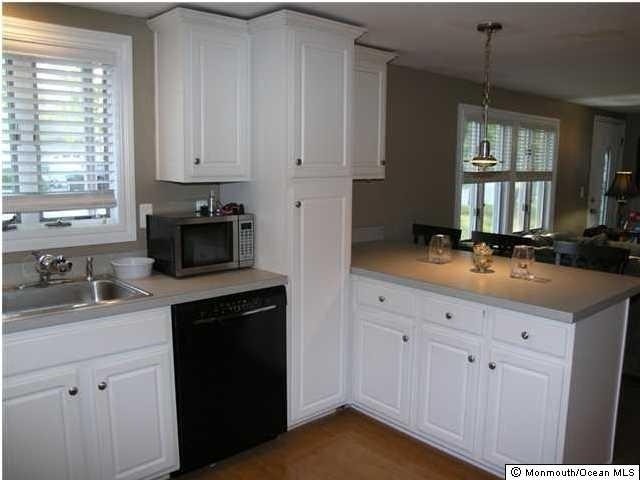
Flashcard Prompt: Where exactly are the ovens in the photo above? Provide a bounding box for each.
[171,286,287,473]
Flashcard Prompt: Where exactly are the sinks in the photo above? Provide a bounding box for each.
[3,276,152,319]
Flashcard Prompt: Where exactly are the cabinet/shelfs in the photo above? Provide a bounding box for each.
[413,275,640,480]
[216,181,354,431]
[350,266,420,438]
[2,297,180,480]
[246,7,370,181]
[350,43,400,180]
[145,6,251,185]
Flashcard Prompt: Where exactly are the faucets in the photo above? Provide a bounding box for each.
[37,252,74,287]
[84,256,95,282]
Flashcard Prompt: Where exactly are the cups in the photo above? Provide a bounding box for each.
[427,234,452,263]
[510,245,535,281]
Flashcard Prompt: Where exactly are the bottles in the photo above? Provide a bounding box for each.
[208,189,216,216]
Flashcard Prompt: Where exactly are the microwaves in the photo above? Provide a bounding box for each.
[146,214,256,277]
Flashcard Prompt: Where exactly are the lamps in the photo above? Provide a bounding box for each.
[462,21,504,168]
[604,170,640,230]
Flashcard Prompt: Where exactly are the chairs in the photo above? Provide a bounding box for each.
[412,223,463,250]
[551,239,631,275]
[470,229,536,260]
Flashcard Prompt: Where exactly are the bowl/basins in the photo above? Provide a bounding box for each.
[110,257,155,279]
